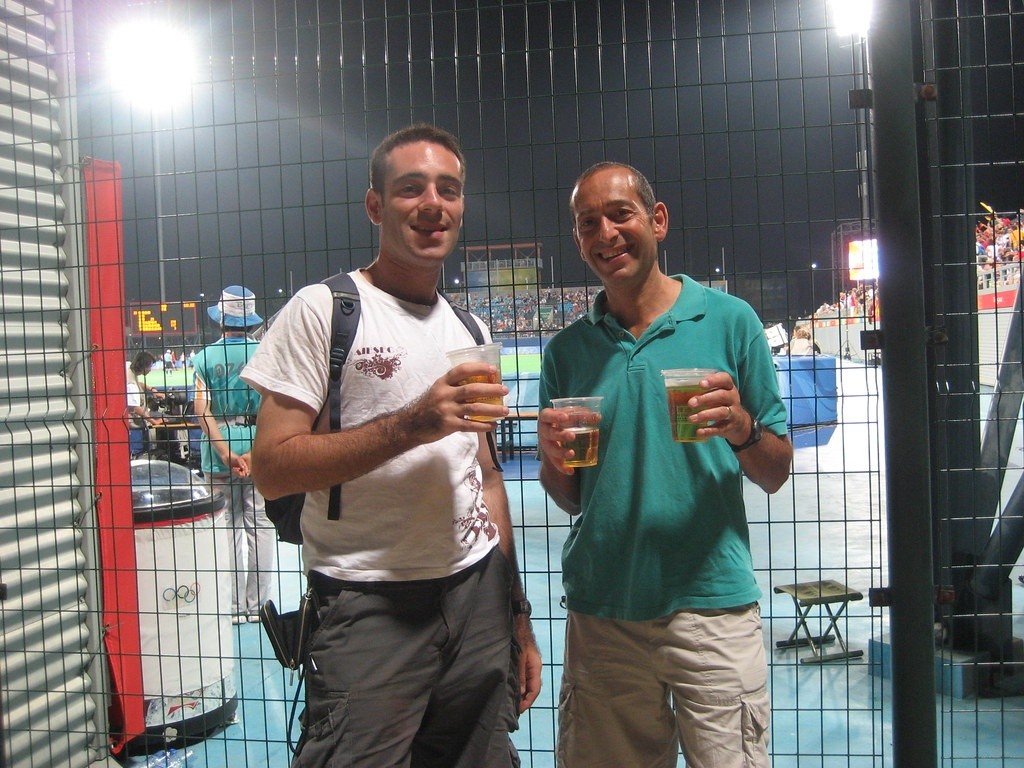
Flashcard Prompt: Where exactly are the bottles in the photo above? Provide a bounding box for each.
[131,748,178,768]
[153,749,194,768]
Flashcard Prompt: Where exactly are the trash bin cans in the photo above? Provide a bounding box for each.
[127,458,241,757]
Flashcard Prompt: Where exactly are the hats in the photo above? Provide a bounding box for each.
[207,286,263,327]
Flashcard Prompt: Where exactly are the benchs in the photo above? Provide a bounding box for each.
[498,411,540,460]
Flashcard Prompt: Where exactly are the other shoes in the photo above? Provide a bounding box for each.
[232,613,261,624]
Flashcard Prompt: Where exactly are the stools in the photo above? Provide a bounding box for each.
[773,579,863,663]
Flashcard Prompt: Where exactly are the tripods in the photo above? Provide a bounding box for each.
[833,289,864,362]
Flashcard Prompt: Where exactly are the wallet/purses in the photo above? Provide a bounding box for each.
[261,592,312,687]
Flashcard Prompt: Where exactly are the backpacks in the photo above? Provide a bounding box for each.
[263,269,504,544]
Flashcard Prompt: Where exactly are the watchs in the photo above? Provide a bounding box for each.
[514,598,533,617]
[725,415,762,452]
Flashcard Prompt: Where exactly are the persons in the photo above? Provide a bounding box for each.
[123,213,1024,623]
[238,123,544,768]
[538,163,794,768]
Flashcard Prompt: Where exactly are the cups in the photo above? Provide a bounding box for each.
[661,368,719,443]
[550,396,604,468]
[447,342,506,422]
